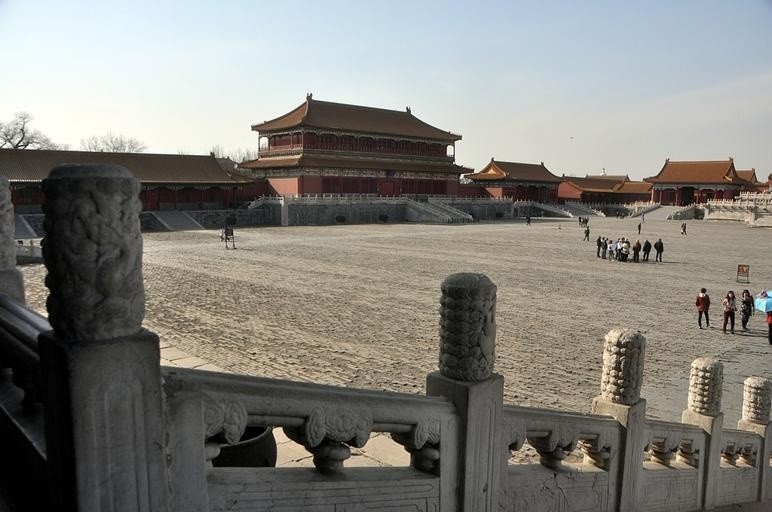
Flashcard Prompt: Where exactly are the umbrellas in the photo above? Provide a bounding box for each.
[755,290,772,314]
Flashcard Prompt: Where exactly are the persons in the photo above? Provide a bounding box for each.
[766,311,772,346]
[619,212,622,218]
[637,222,642,235]
[740,289,754,332]
[524,213,533,226]
[578,215,590,242]
[695,287,711,329]
[680,223,687,235]
[616,211,620,219]
[680,223,684,233]
[595,236,665,264]
[642,213,645,222]
[721,290,738,334]
[620,212,625,219]
